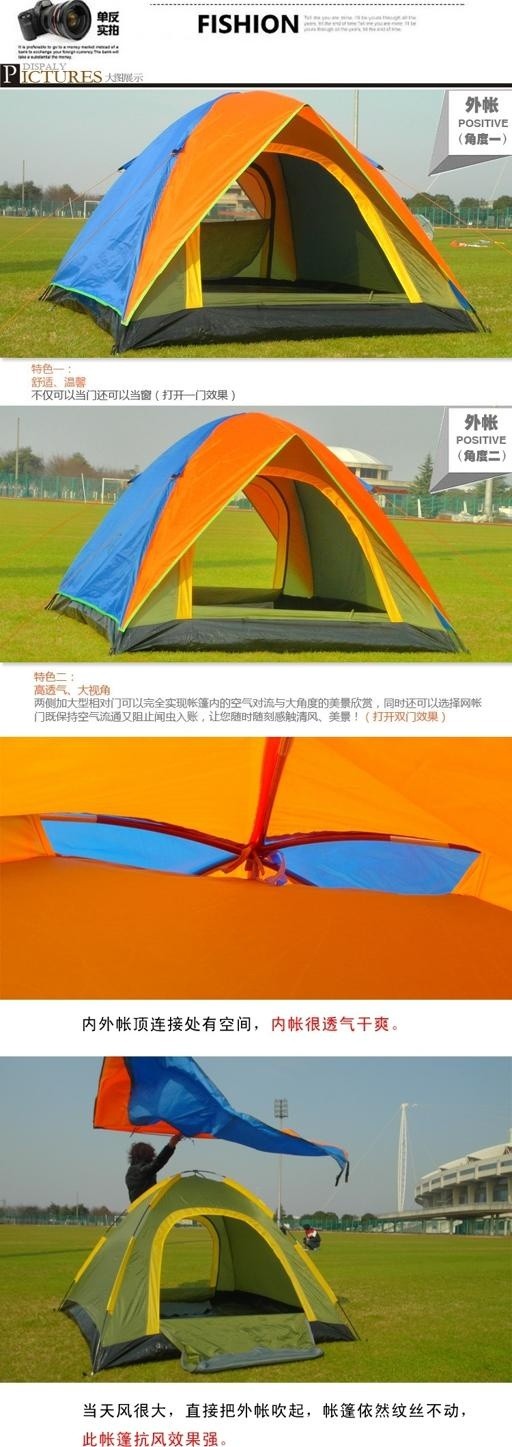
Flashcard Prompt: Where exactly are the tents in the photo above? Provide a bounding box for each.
[41,409,471,659]
[39,89,490,353]
[58,1168,361,1375]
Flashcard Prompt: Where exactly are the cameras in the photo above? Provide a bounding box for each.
[18,0,92,41]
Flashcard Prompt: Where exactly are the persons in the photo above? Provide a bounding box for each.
[125,1133,183,1203]
[302,1222,322,1250]
